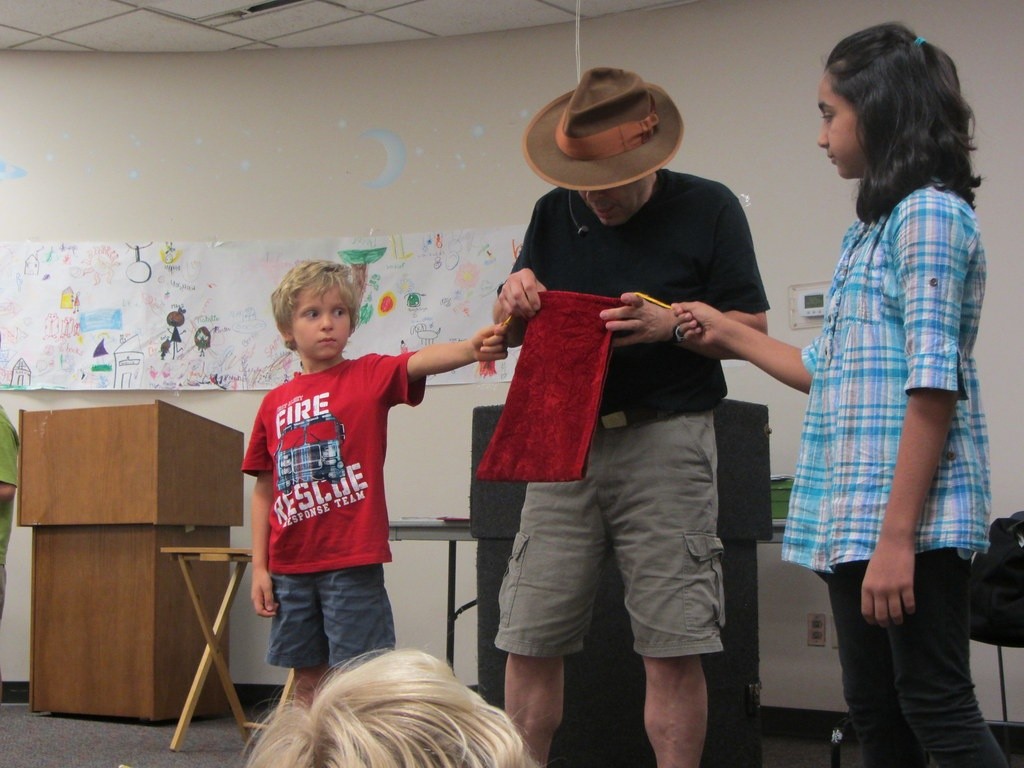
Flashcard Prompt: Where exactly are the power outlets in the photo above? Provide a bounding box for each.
[807,613,826,646]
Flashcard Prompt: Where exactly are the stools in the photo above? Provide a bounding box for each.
[159,547,298,754]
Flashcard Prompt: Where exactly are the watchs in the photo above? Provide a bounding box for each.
[669,325,685,345]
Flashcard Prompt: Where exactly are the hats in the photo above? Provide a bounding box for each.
[522,67,683,190]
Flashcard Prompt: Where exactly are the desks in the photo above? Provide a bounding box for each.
[388,516,787,677]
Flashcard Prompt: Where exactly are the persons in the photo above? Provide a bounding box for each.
[670,21,1010,767]
[242,260,508,705]
[493,65,770,768]
[240,643,566,768]
